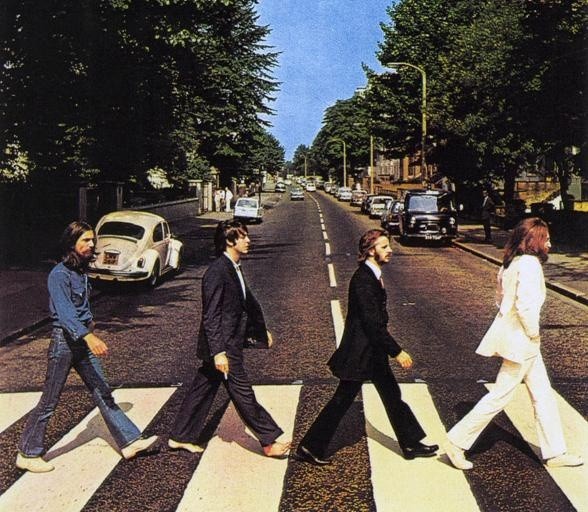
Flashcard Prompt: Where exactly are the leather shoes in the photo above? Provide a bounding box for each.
[295,444,331,466]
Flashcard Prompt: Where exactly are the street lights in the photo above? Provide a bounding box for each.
[353,121,374,195]
[332,138,347,188]
[386,61,427,186]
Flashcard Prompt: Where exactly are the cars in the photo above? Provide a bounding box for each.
[274,181,287,193]
[289,187,305,200]
[380,200,404,232]
[368,196,393,218]
[277,177,283,183]
[292,176,339,197]
[234,196,263,223]
[400,191,457,244]
[87,209,182,288]
[350,189,368,205]
[285,180,292,185]
[361,196,377,214]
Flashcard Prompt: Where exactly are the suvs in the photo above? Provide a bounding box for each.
[339,187,352,200]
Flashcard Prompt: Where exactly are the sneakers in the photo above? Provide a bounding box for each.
[547,456,585,466]
[442,438,473,470]
[401,444,439,460]
[14,454,55,472]
[120,436,158,460]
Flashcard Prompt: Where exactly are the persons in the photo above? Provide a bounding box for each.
[361,191,368,215]
[292,227,439,468]
[439,215,585,471]
[165,217,293,460]
[224,186,233,212]
[218,186,225,212]
[14,220,159,475]
[480,189,495,243]
[212,188,220,212]
